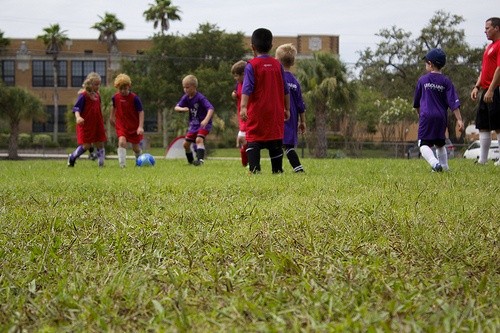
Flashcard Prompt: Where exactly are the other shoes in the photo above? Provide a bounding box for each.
[68,153,75,167]
[193,158,205,166]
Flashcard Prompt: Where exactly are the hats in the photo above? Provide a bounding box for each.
[422,48,446,66]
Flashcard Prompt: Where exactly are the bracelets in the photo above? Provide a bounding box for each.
[472,85,480,91]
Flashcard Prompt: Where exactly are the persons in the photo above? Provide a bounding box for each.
[274,43,306,173]
[232,60,248,167]
[412,47,465,173]
[109,73,144,169]
[174,75,214,166]
[67,72,108,167]
[470,17,500,166]
[239,28,290,175]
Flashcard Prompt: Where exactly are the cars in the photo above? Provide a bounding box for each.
[463,139,500,160]
[406,136,455,159]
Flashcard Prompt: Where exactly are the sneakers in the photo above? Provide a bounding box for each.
[432,163,443,173]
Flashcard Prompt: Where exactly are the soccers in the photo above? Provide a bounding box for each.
[135,152,155,167]
